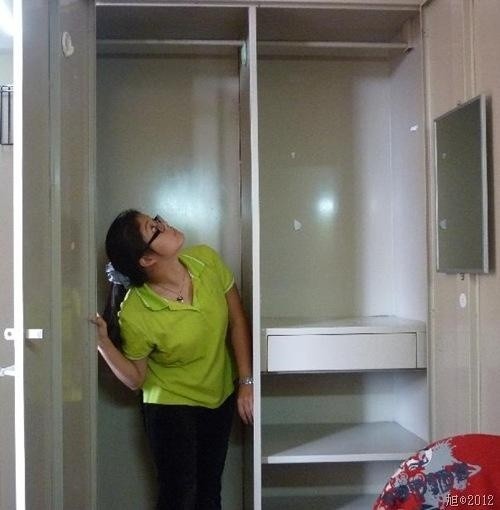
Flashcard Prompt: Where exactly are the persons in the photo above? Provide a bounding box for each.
[89,210,253,510]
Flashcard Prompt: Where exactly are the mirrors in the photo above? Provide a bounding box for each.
[427,92,491,277]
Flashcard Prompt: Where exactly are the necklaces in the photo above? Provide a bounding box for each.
[157,269,190,306]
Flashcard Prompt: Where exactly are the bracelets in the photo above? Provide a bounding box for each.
[237,376,254,384]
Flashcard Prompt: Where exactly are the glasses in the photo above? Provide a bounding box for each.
[145,214,166,249]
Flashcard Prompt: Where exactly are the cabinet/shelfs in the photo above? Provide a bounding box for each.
[94,1,431,510]
